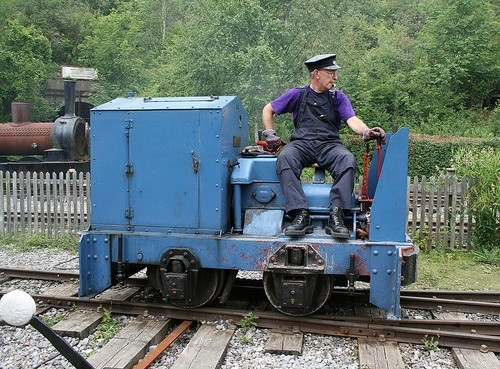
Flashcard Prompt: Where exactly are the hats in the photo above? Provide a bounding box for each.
[304,54,342,72]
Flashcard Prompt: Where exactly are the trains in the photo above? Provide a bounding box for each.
[0,79,91,178]
[78,91,419,321]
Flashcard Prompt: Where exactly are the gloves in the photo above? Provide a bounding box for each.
[363,127,386,145]
[262,129,281,150]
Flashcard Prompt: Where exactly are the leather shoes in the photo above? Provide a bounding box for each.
[285,209,313,237]
[325,207,351,239]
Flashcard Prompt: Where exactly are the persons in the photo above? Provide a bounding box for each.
[262,54,386,239]
